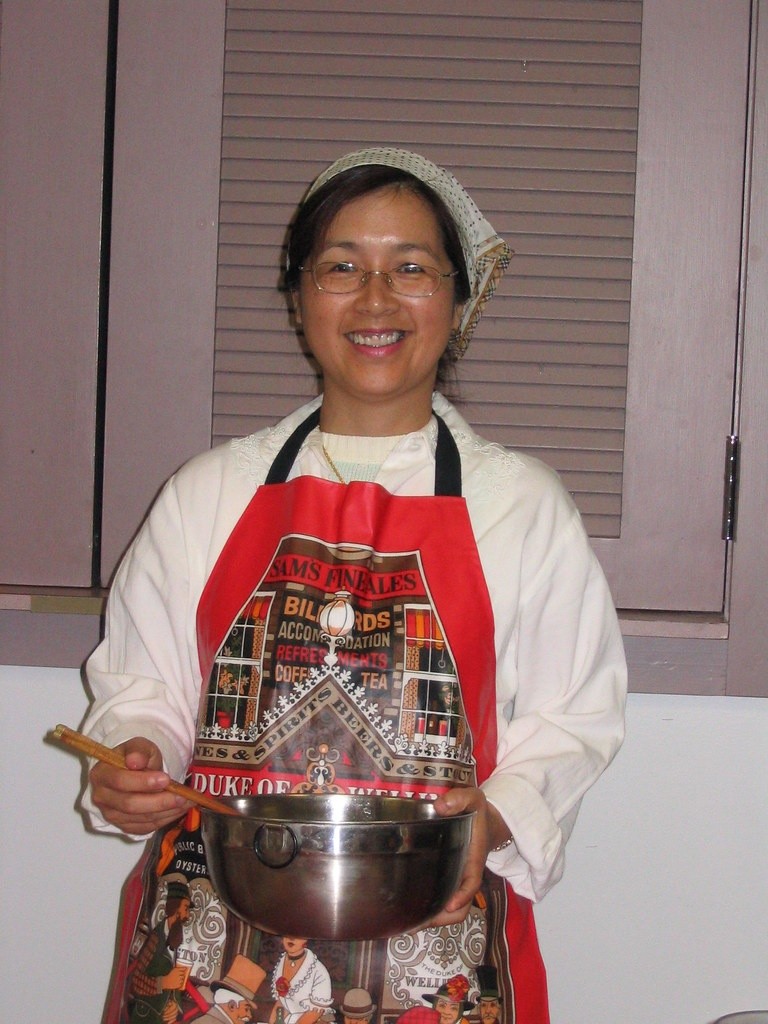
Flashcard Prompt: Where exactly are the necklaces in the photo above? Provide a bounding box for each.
[321,443,348,485]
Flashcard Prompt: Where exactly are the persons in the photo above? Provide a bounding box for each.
[80,148,628,1024]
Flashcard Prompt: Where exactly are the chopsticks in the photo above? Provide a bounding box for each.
[52,724,243,817]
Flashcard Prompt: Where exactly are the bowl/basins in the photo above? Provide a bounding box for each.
[197,793,478,942]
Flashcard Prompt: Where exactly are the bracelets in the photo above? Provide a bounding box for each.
[492,835,513,852]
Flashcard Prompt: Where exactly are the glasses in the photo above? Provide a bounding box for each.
[298,261,459,298]
